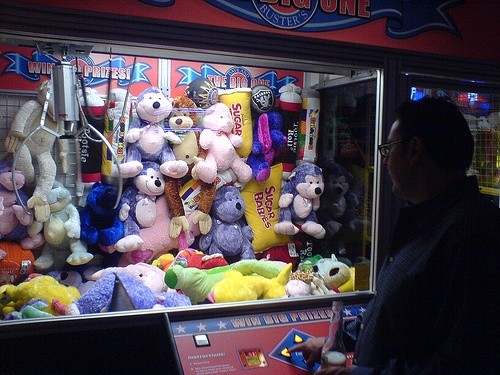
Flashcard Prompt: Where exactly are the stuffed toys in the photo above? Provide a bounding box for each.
[0,79,355,321]
[463,112,500,189]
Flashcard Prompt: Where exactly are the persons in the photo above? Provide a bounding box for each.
[287,95,500,375]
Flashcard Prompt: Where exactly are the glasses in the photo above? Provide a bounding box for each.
[378,138,406,157]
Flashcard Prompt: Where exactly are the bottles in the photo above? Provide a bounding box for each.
[320,300,347,370]
